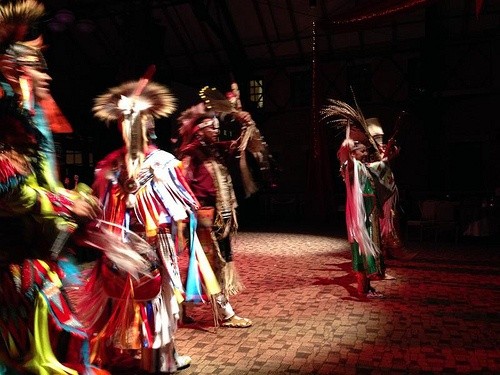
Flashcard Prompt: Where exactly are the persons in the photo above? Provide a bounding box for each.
[0,0,269,375]
[322,101,398,299]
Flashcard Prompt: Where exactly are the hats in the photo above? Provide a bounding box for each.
[93,64,177,179]
[0,0,47,83]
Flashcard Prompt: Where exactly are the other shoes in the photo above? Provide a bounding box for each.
[384,273,397,280]
[160,352,191,372]
[221,316,252,327]
[367,287,383,298]
